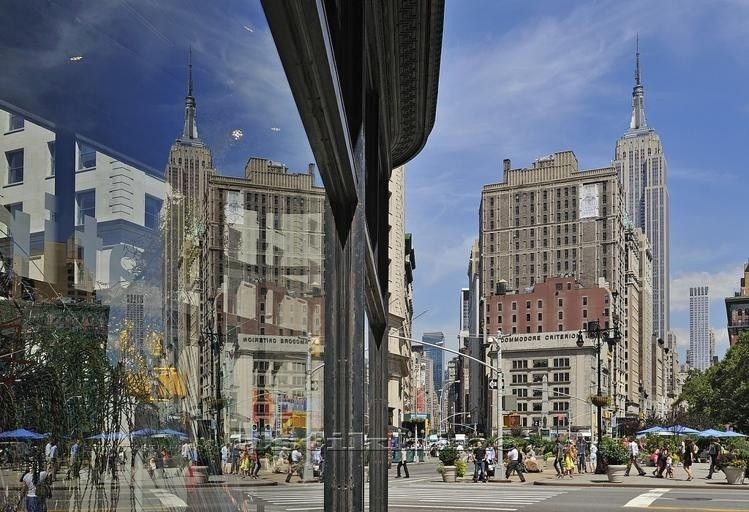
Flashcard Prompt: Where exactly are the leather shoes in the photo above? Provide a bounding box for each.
[403,476,409,478]
[395,476,401,478]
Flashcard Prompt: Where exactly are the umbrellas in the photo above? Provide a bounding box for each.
[634,423,749,442]
[0,424,193,444]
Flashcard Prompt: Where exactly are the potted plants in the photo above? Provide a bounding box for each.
[715,451,749,485]
[598,436,632,483]
[188,437,219,484]
[438,446,460,484]
[591,394,612,409]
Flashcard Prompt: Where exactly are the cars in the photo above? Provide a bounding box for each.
[410,433,488,457]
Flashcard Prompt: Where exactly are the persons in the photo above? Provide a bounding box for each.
[395,443,410,478]
[3,438,194,512]
[552,434,598,480]
[618,435,727,481]
[196,437,327,483]
[473,440,525,482]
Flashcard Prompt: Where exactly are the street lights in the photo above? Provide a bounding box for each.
[439,380,461,437]
[576,320,622,473]
[223,312,273,443]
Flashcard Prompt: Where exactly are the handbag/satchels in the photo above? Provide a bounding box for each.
[35,482,52,499]
